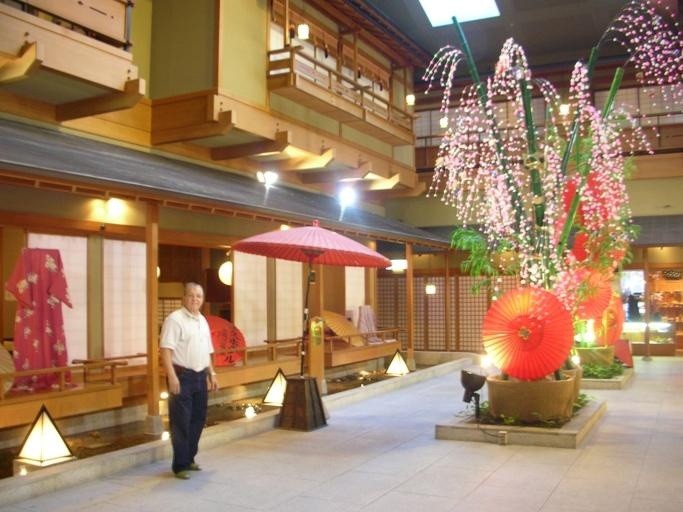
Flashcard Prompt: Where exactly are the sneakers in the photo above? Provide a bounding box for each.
[189,463,202,470]
[176,470,190,478]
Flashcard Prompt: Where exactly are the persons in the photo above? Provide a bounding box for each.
[159,282,218,480]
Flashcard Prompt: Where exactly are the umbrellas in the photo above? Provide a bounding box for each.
[231,219,392,376]
[321,309,365,347]
[481,286,574,381]
[205,315,245,367]
[579,271,612,320]
[0,343,16,396]
[595,293,626,347]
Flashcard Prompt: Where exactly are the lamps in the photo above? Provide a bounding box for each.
[384,347,410,375]
[260,366,288,405]
[216,260,231,286]
[15,403,72,463]
[459,368,484,421]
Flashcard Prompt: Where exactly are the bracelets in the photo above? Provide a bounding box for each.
[208,372,217,376]
